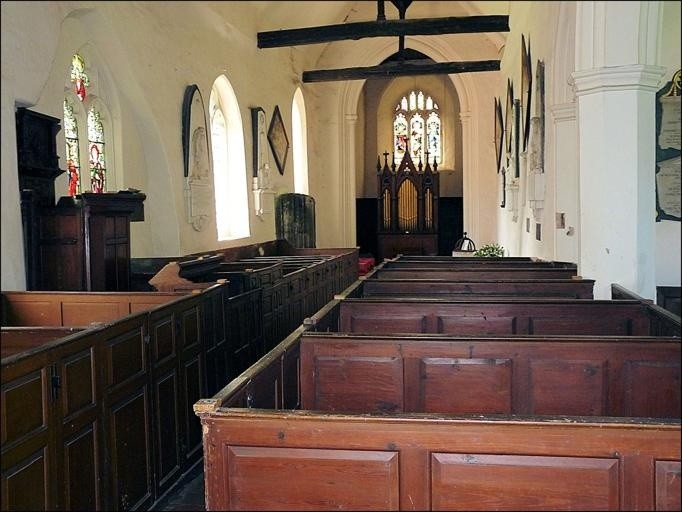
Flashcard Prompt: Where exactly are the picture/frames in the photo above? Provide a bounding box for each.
[267,104,290,176]
[493,33,532,174]
